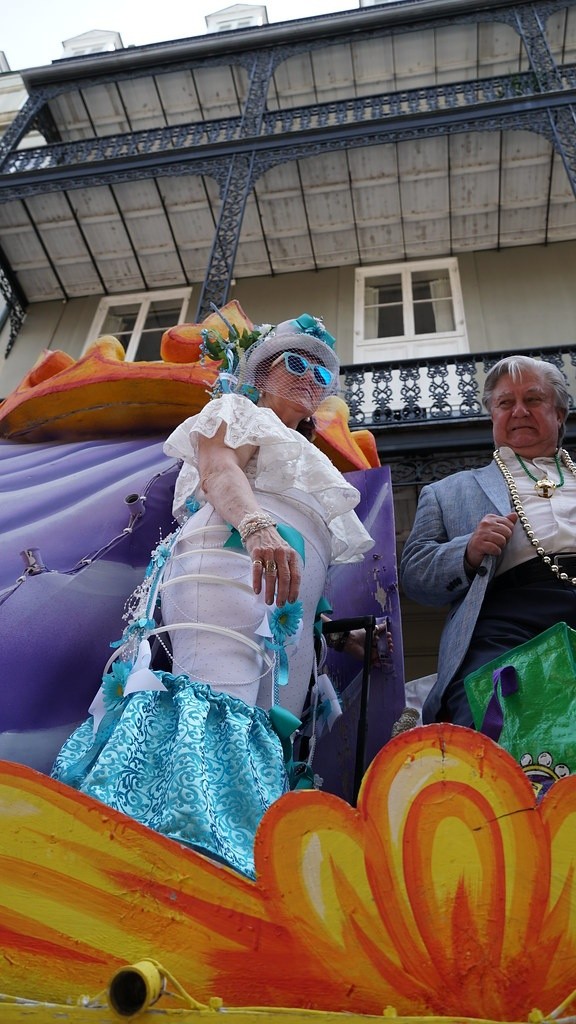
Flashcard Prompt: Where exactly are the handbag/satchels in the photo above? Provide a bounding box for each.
[463,623,576,803]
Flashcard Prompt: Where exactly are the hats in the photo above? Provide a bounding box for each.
[247,314,339,401]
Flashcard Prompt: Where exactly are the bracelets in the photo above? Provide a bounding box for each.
[238,512,277,546]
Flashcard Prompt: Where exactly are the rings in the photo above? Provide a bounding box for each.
[266,559,276,571]
[254,560,262,563]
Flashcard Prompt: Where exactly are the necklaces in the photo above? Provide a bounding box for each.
[515,453,564,498]
[493,448,576,585]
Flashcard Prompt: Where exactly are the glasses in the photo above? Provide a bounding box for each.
[269,352,334,388]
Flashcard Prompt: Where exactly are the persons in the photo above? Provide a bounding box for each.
[400,354,576,745]
[52,314,376,884]
